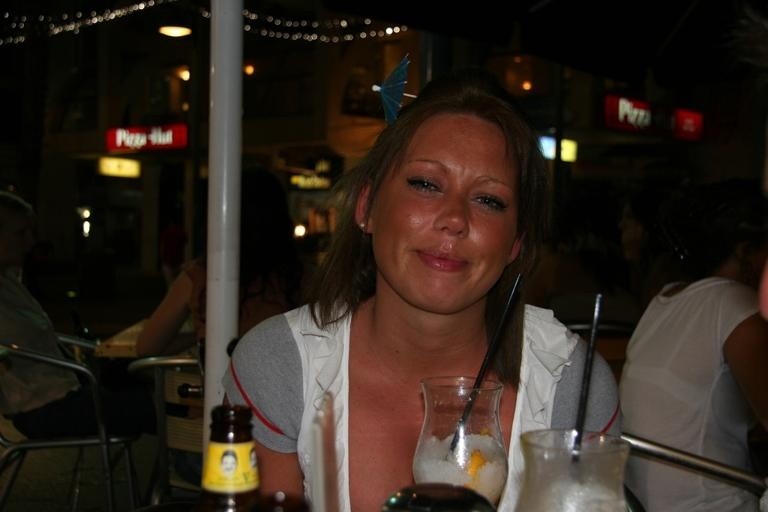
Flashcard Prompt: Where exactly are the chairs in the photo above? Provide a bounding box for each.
[0,334,204,509]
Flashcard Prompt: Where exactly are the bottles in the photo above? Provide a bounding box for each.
[200,403,261,512]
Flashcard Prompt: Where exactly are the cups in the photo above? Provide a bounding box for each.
[379,374,634,511]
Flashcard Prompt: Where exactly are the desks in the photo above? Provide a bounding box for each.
[94,319,196,362]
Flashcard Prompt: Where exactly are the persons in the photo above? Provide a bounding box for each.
[134,237,293,361]
[220,78,622,512]
[615,178,767,512]
[1,190,205,511]
[540,174,669,327]
